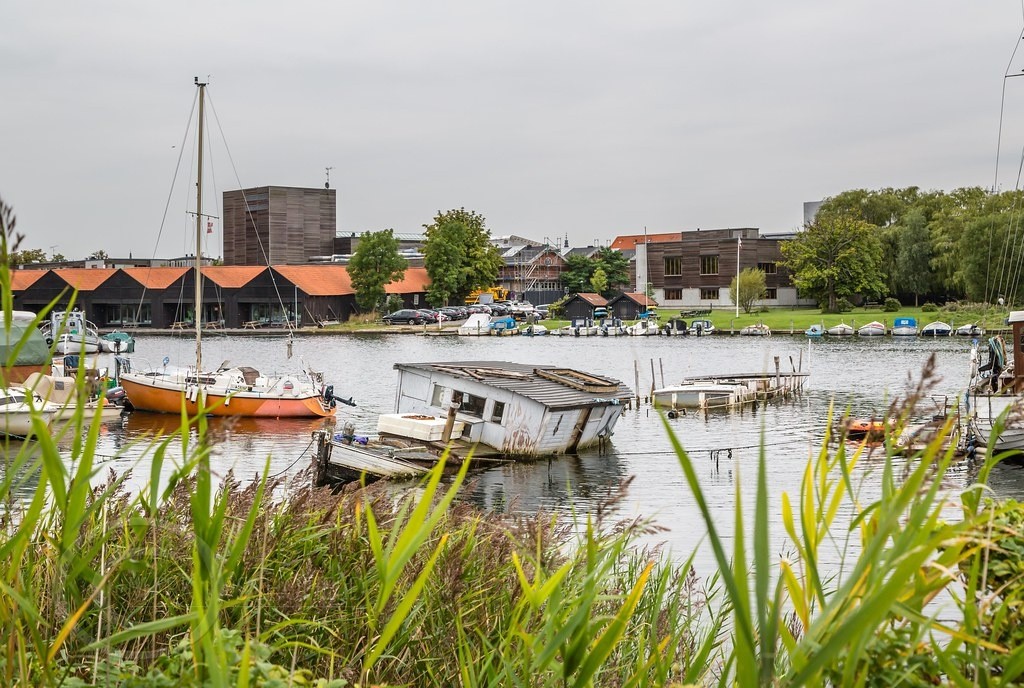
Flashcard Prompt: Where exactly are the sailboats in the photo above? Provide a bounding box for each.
[626,278,660,335]
[965,0,1024,459]
[120,75,356,418]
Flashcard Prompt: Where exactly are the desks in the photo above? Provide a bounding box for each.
[205,322,219,330]
[244,321,262,329]
[283,321,295,329]
[172,322,189,329]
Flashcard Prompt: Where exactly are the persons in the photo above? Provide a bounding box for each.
[998,296,1004,305]
[532,310,540,324]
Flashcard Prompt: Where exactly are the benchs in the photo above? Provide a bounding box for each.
[242,324,262,326]
[170,325,187,327]
[204,324,221,326]
[122,323,139,328]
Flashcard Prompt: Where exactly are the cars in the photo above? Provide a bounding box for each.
[418,300,554,324]
[381,309,425,325]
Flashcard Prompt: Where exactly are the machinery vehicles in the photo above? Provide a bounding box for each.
[465,286,509,306]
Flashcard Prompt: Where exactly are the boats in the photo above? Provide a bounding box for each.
[458,313,494,336]
[312,361,636,482]
[740,323,770,335]
[40,306,100,356]
[661,319,687,336]
[827,323,853,335]
[688,319,715,336]
[956,323,981,335]
[491,318,520,336]
[858,321,884,336]
[522,325,547,336]
[921,320,951,336]
[831,417,897,441]
[0,311,131,442]
[805,324,823,336]
[549,317,627,336]
[99,327,135,354]
[883,395,967,457]
[891,317,917,336]
